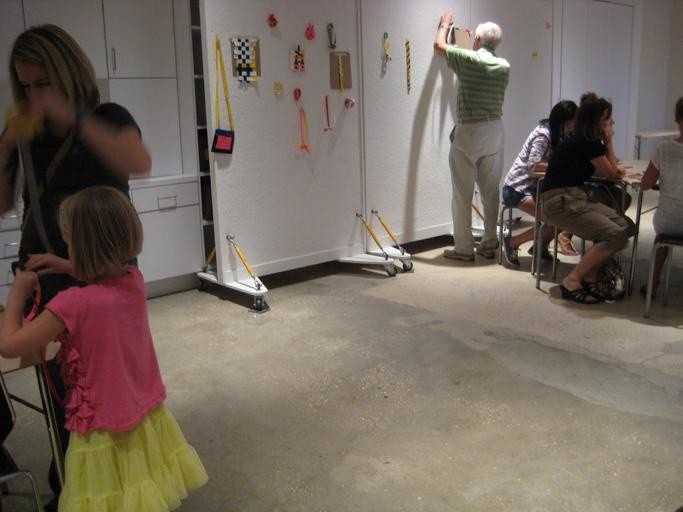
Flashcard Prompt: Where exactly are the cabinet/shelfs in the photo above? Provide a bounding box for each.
[24,2,183,177]
[0,2,35,204]
[127,178,204,303]
[1,197,24,308]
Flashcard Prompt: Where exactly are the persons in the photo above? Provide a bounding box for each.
[0,184,208,512]
[434,11,511,261]
[640,96,682,297]
[501,100,580,269]
[0,22,153,511]
[539,91,641,304]
[556,230,579,256]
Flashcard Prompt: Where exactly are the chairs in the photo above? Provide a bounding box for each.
[0,375,40,512]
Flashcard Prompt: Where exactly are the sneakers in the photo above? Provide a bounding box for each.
[444,250,474,261]
[476,245,495,259]
[502,236,520,268]
[528,246,560,264]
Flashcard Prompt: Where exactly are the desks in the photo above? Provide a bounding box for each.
[0,341,67,492]
[531,161,660,297]
[635,130,680,160]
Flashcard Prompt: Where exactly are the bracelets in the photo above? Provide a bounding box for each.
[438,23,452,32]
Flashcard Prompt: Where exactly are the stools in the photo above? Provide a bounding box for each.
[499,202,515,266]
[536,219,586,293]
[643,234,683,318]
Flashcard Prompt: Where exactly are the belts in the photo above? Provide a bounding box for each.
[457,117,497,124]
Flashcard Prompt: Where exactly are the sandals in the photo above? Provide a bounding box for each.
[557,234,580,256]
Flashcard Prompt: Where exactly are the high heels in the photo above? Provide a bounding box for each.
[560,285,598,305]
[582,281,607,303]
